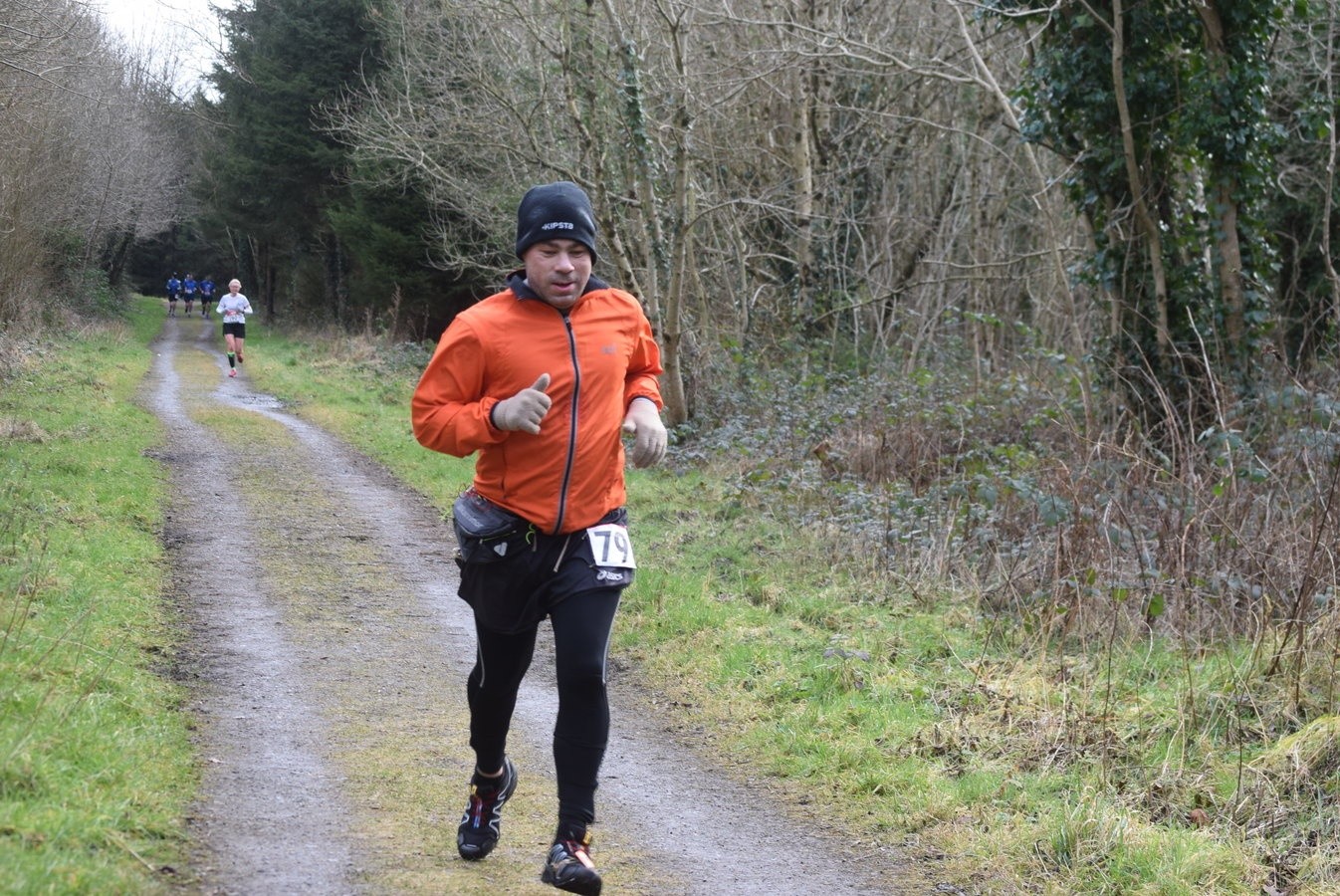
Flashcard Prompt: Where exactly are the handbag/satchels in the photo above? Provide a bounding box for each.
[454,493,523,563]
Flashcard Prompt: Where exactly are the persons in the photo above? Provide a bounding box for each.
[166,271,216,320]
[411,178,669,895]
[215,279,253,377]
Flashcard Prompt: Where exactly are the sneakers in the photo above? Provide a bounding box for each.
[541,831,602,896]
[457,754,517,860]
[236,353,243,364]
[229,368,236,377]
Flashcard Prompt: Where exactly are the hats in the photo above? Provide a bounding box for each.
[515,181,598,266]
